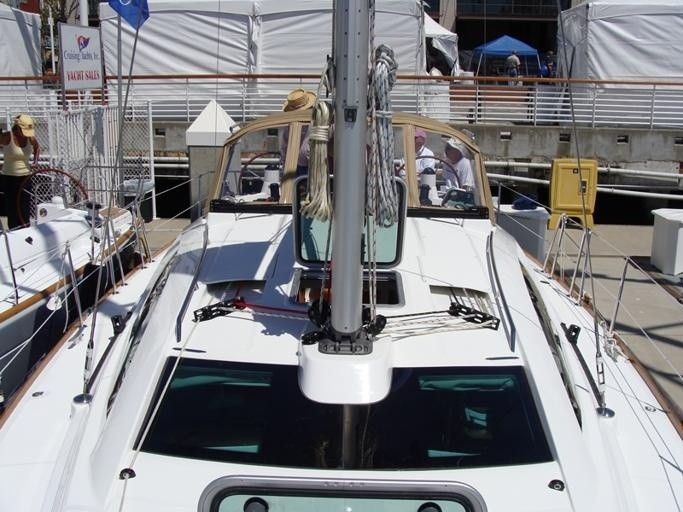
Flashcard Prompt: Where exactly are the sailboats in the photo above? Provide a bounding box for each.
[1,1,683,511]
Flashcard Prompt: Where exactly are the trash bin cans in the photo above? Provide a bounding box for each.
[650,208,683,276]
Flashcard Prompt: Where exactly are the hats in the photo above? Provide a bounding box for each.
[415,130,426,138]
[15,114,35,138]
[442,138,469,157]
[283,89,317,113]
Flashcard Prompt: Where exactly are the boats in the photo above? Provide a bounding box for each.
[1,168,145,417]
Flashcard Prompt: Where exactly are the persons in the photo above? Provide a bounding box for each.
[398,128,438,199]
[425,55,444,81]
[278,89,340,202]
[536,51,558,86]
[439,136,474,199]
[504,50,521,86]
[0,114,40,229]
